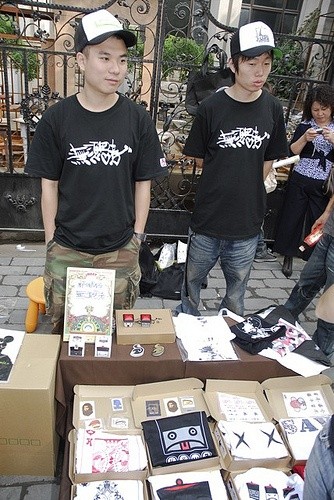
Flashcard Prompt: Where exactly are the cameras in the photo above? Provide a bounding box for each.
[315,128,326,134]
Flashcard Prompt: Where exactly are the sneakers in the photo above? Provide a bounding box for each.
[253,252,277,262]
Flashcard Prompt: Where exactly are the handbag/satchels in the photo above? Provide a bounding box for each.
[219,307,286,355]
[186,44,235,115]
[139,240,188,300]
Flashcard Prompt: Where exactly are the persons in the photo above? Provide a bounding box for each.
[303,415,334,500]
[29,10,155,335]
[279,83,334,277]
[180,21,289,327]
[280,193,334,362]
[250,76,276,264]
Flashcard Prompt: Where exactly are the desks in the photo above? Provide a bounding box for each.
[178,314,300,382]
[55,321,186,407]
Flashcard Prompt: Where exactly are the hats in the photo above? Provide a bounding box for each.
[74,10,137,53]
[226,21,283,63]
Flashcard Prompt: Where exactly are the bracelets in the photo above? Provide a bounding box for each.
[134,233,147,242]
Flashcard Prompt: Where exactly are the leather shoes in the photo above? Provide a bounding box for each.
[282,255,293,279]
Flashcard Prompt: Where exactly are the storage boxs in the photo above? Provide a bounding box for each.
[116,309,177,347]
[68,374,334,500]
[0,334,62,477]
